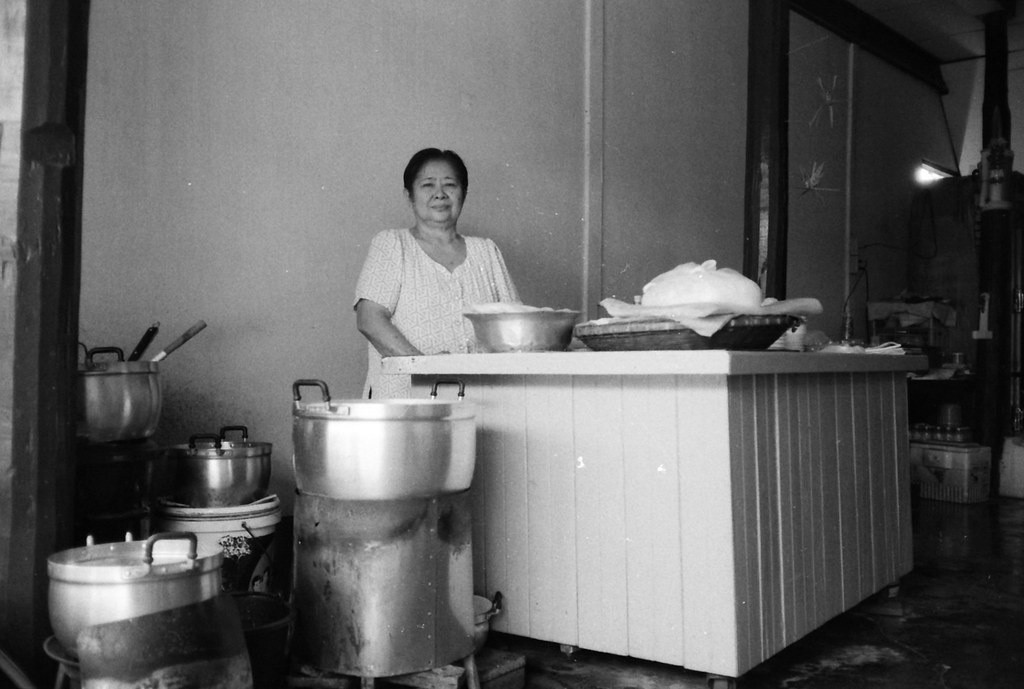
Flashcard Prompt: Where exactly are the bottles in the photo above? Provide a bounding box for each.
[912,423,972,443]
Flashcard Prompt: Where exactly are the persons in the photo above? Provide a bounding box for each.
[353,148,522,402]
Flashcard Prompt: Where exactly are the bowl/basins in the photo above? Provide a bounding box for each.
[43,635,80,682]
[463,311,581,353]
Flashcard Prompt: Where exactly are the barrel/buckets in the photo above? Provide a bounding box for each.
[222,592,296,689]
[151,496,283,594]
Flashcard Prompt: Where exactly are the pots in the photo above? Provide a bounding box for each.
[74,341,162,442]
[47,514,224,658]
[474,591,502,655]
[291,375,478,501]
[167,426,273,508]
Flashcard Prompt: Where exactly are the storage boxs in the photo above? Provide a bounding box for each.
[868,301,951,349]
